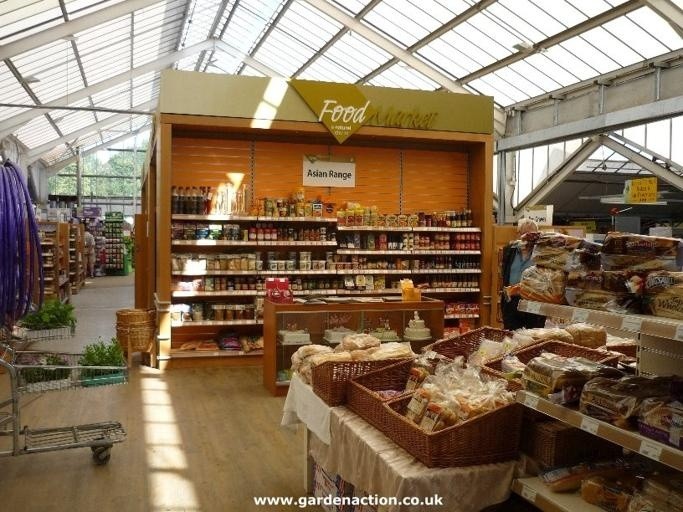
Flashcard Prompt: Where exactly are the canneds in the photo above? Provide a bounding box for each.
[267,251,297,271]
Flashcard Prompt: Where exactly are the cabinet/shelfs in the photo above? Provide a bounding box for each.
[70,223,85,294]
[24,221,72,304]
[104,211,124,276]
[171,213,682,507]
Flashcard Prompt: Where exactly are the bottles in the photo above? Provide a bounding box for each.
[171,185,213,214]
[446,208,472,228]
[431,255,480,287]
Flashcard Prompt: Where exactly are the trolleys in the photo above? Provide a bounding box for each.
[0,342,129,467]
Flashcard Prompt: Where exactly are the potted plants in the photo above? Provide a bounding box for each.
[12,299,78,340]
[20,354,73,393]
[77,336,126,387]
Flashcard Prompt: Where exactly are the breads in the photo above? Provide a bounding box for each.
[404,366,507,432]
[544,460,683,511]
[522,231,683,319]
[290,333,412,386]
[522,351,683,451]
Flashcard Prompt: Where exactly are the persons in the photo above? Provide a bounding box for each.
[499,218,550,332]
